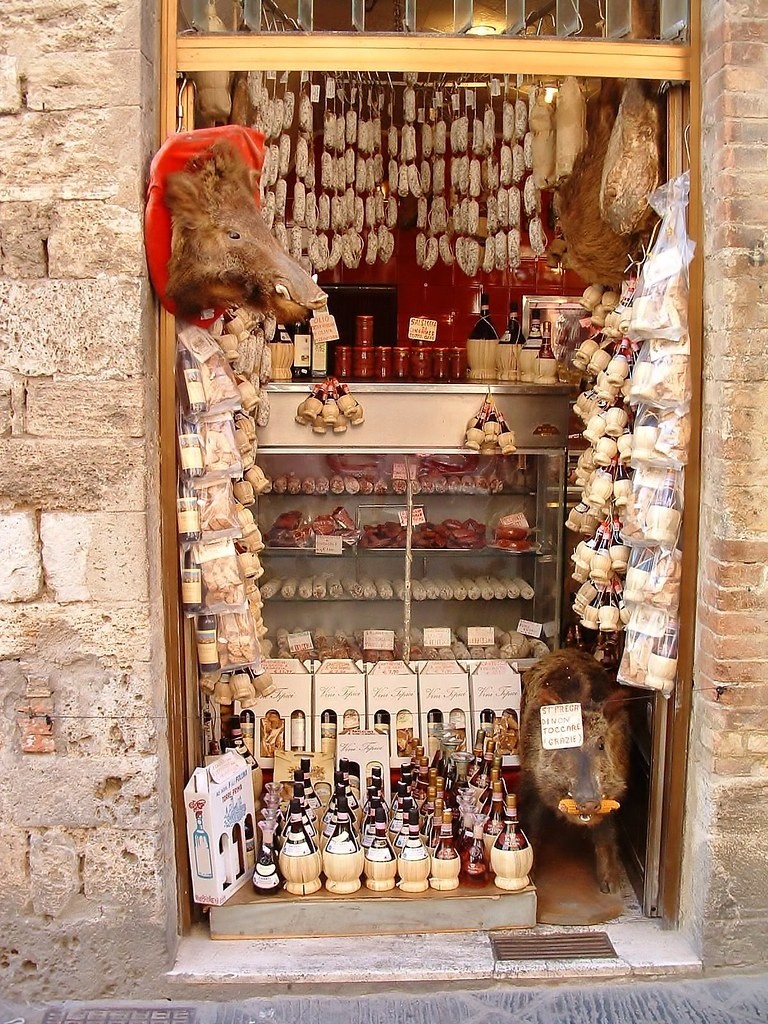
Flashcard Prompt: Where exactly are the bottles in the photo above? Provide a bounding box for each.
[520,309,543,382]
[534,321,559,385]
[178,303,270,707]
[562,268,686,695]
[204,708,535,892]
[294,378,365,436]
[496,302,526,381]
[465,293,501,378]
[464,394,517,457]
[270,323,296,380]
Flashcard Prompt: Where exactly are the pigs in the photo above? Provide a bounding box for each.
[161,139,329,327]
[515,645,636,897]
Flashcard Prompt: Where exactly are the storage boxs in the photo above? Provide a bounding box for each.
[182,750,261,906]
[253,659,523,809]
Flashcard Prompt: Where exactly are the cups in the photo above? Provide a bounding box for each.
[292,316,312,377]
[312,334,327,377]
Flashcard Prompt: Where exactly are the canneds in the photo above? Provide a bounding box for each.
[354,315,374,346]
[411,347,433,380]
[392,347,411,380]
[451,347,468,380]
[354,346,375,379]
[334,345,353,379]
[374,347,394,380]
[432,347,451,380]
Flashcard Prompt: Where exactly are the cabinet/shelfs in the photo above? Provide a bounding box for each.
[257,388,590,663]
